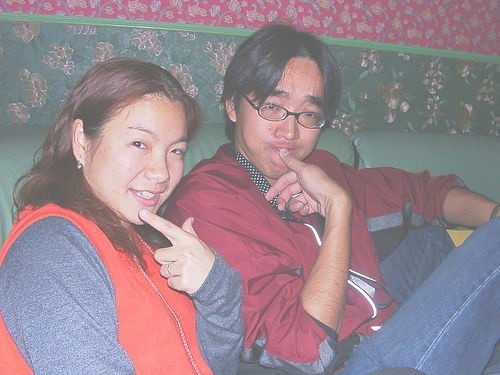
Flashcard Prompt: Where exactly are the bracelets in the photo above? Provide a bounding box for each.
[489,205,500,219]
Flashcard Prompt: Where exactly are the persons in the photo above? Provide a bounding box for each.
[156,24,500,375]
[0,57,243,375]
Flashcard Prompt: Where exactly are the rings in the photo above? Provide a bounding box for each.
[167,264,172,276]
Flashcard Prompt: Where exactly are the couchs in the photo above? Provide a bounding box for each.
[0,119,500,255]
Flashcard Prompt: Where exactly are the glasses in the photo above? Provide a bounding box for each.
[240,91,328,129]
